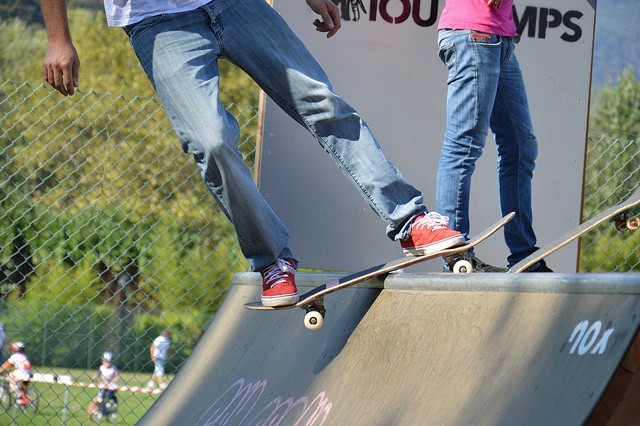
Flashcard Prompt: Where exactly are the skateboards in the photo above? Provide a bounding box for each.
[508,186,640,273]
[243,212,516,331]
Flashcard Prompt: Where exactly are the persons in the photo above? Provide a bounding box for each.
[83,351,121,420]
[434,0,555,273]
[0,342,34,406]
[146,329,173,396]
[38,0,465,306]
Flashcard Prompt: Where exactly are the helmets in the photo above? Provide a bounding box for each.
[11,342,25,352]
[102,352,113,361]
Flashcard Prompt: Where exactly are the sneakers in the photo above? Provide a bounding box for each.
[16,399,28,404]
[260,259,300,307]
[400,211,466,256]
[454,252,509,272]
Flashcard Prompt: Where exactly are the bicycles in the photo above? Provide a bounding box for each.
[87,380,118,422]
[0,373,38,415]
